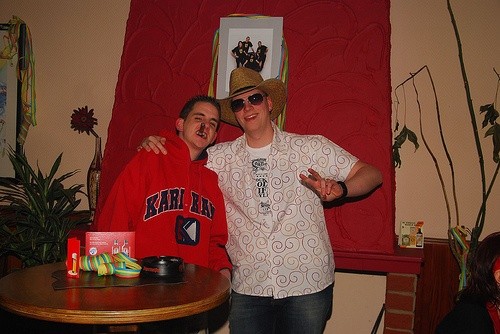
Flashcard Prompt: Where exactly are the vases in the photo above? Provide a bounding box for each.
[87,136,103,220]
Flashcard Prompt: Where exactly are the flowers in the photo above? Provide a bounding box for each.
[70,106,100,137]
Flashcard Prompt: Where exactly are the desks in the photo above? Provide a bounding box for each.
[0,260,231,334]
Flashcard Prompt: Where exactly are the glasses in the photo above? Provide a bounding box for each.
[231,93,267,112]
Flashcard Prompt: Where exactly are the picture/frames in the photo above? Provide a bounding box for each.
[216,16,282,99]
[0,22,26,187]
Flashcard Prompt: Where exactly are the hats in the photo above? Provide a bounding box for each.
[213,68,287,129]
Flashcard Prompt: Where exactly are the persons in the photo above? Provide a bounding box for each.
[432,231,500,334]
[231,36,269,71]
[137,66,383,334]
[98,96,233,334]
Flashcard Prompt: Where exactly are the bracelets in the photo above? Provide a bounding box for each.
[337,181,348,197]
